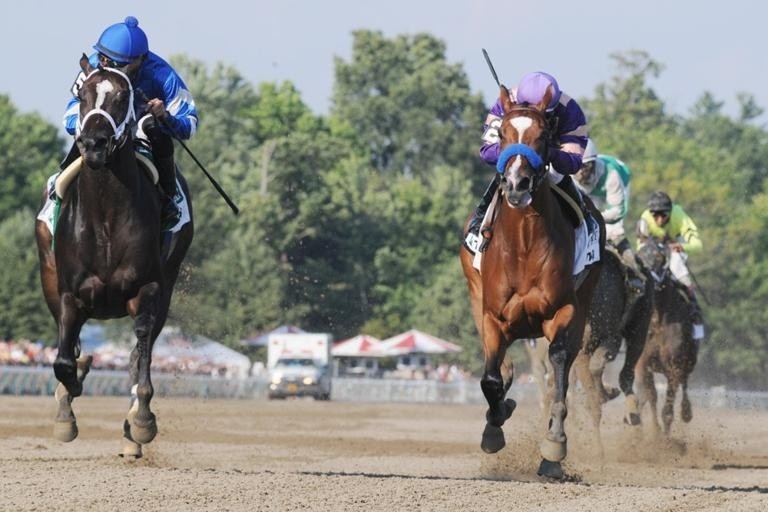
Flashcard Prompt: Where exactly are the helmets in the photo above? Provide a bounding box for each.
[93,16,148,63]
[583,138,596,162]
[518,71,560,112]
[647,192,672,213]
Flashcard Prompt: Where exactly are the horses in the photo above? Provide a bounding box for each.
[34,50,194,459]
[612,229,700,452]
[523,245,631,474]
[460,83,608,481]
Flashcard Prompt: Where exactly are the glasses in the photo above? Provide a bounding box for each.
[97,53,127,67]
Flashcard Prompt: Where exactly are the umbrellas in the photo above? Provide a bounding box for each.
[333,331,384,357]
[367,327,463,359]
[238,324,305,347]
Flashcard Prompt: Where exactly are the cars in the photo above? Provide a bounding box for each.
[267,352,333,402]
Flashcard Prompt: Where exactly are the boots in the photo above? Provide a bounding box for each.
[683,282,701,325]
[151,154,181,234]
[617,239,642,289]
[467,174,501,253]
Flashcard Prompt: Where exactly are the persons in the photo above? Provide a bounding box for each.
[460,70,607,279]
[1,336,265,397]
[571,139,648,296]
[45,15,200,232]
[343,360,584,407]
[634,191,707,339]
